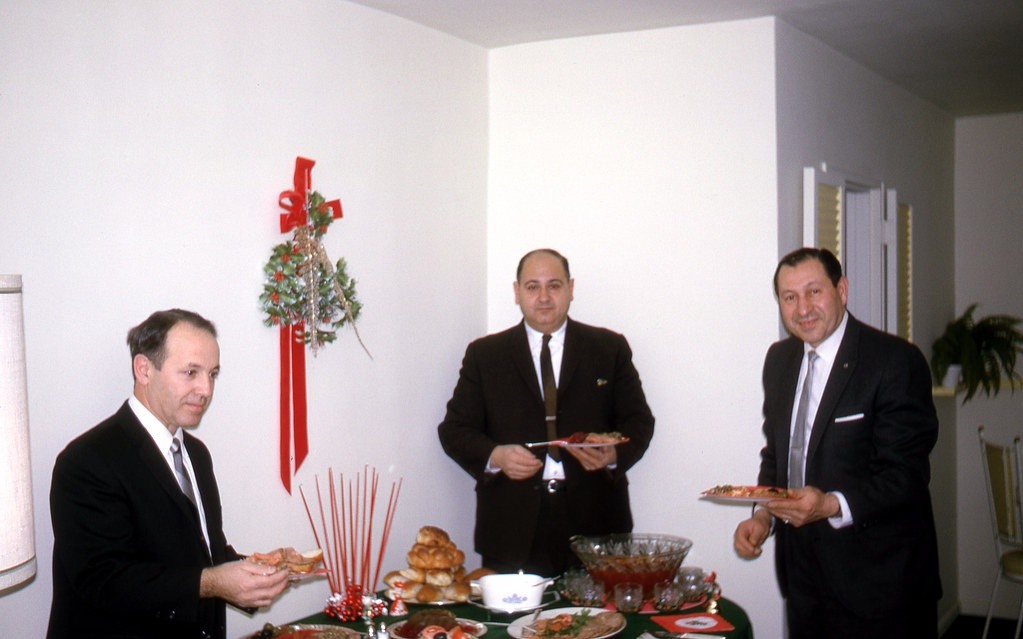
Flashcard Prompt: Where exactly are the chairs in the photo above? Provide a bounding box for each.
[978,424,1023,639]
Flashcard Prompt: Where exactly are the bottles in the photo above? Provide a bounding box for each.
[364,622,390,639]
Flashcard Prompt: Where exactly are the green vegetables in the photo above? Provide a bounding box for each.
[536,608,594,638]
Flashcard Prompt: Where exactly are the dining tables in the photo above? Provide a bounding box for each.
[242,593,756,639]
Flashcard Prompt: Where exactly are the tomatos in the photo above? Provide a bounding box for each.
[421,624,470,639]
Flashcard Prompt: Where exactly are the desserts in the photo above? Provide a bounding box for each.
[399,608,460,639]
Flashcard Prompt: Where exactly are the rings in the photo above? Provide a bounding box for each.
[784,518,789,525]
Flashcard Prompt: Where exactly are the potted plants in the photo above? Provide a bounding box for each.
[930,300,1023,408]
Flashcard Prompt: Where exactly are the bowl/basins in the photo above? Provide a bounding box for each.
[570,535,691,598]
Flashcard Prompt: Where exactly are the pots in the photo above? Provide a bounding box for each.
[469,574,554,613]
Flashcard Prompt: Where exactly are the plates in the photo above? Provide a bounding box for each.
[385,618,487,639]
[383,571,481,605]
[506,606,625,639]
[560,441,613,447]
[278,623,361,639]
[702,491,775,502]
[565,592,707,613]
[287,568,330,582]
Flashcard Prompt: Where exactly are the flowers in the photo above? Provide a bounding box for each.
[259,191,360,346]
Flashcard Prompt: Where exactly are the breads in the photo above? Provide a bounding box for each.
[387,525,497,601]
[281,549,324,574]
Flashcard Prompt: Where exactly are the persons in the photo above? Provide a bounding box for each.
[47,308,301,639]
[437,249,655,579]
[733,247,943,639]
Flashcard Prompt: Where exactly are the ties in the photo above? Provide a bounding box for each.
[789,350,819,489]
[170,438,198,509]
[539,334,559,463]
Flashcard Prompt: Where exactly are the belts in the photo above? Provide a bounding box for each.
[539,479,564,493]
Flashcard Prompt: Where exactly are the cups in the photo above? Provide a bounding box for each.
[564,566,710,611]
